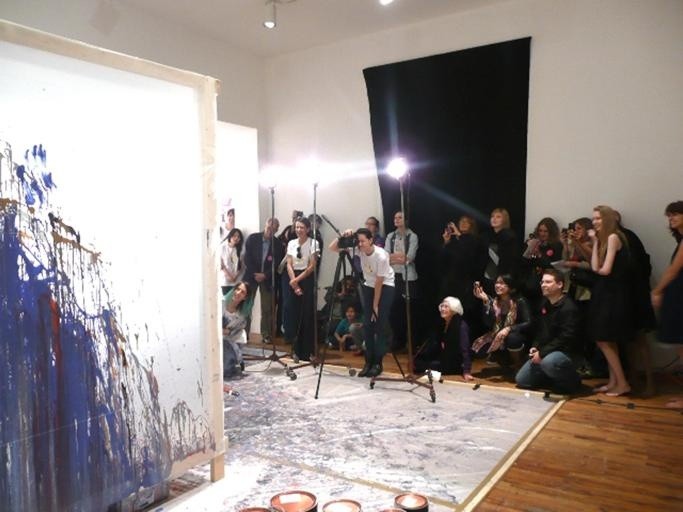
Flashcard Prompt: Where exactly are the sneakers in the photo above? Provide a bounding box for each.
[357,363,383,379]
[262,336,272,345]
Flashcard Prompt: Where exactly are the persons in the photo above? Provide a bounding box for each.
[651,199,683,413]
[221,199,647,398]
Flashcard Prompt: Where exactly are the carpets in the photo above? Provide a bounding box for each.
[119,356,567,512]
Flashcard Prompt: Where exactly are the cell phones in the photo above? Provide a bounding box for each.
[447,225,452,234]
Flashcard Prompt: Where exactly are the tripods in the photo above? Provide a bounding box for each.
[368,271,437,403]
[242,215,295,368]
[314,251,407,401]
[284,222,357,381]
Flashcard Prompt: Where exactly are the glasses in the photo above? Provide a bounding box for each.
[295,246,303,260]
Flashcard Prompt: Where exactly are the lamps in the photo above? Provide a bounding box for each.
[263,0,277,29]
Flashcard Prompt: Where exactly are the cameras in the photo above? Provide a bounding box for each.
[473,281,480,288]
[336,234,358,249]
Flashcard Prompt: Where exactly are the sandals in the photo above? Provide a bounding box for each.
[590,384,621,397]
